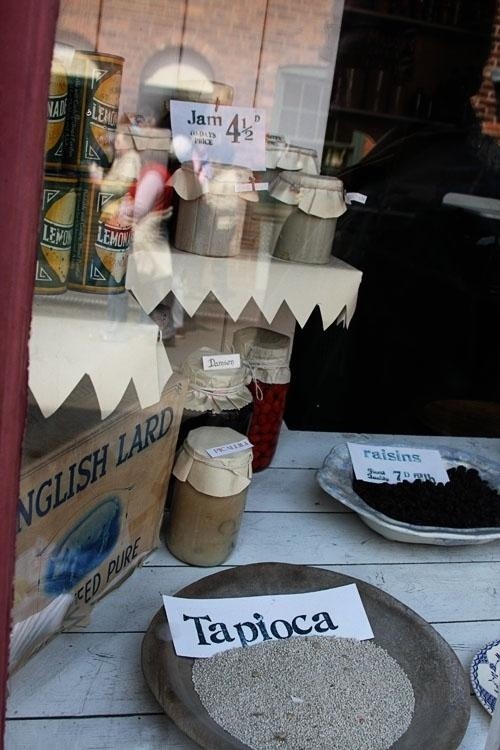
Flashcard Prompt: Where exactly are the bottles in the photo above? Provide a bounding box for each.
[115,78,346,267]
[164,326,291,568]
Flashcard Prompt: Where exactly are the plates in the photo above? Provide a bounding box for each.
[315,439,500,546]
[141,563,472,750]
[470,640,500,717]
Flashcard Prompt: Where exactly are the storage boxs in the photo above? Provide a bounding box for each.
[11,374,192,681]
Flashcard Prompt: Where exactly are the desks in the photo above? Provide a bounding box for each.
[0,424,498,749]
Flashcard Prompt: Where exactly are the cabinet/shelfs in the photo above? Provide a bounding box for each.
[32,245,363,345]
